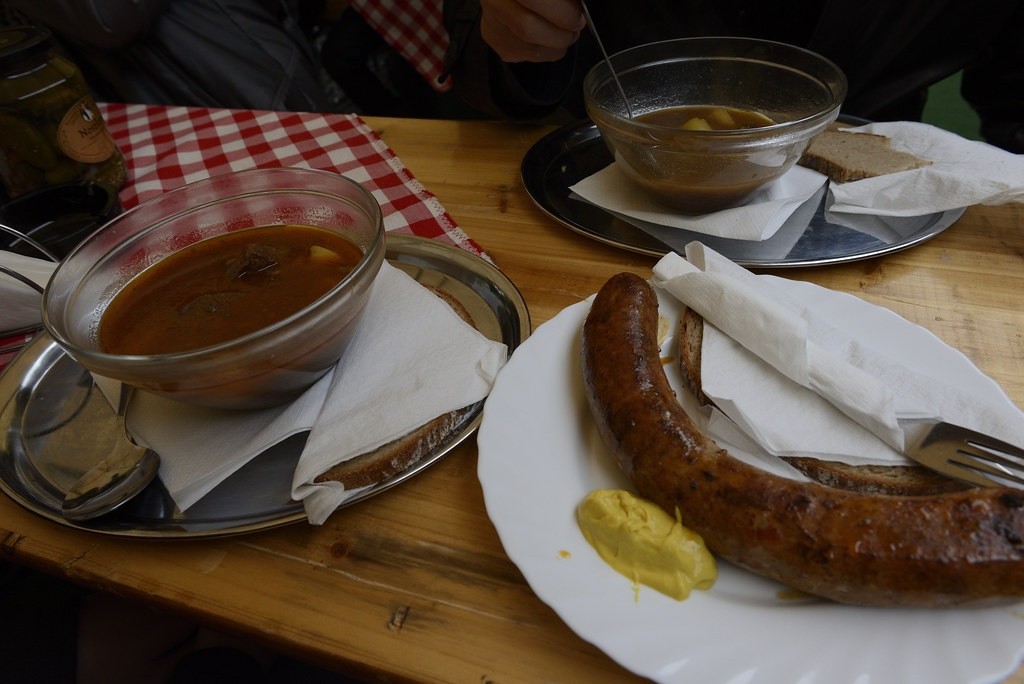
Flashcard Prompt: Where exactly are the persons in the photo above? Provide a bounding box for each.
[0,0,321,111]
[443,0,1024,154]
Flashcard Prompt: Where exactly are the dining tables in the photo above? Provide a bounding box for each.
[0,101,1024,684]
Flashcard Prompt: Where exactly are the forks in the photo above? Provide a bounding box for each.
[897,418,1024,494]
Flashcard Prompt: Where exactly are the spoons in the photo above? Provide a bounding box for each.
[61,379,161,522]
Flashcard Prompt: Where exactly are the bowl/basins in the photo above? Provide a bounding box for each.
[0,180,122,264]
[40,165,387,412]
[582,36,848,212]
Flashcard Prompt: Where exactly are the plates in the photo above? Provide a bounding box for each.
[519,114,967,269]
[0,231,532,541]
[476,273,1024,684]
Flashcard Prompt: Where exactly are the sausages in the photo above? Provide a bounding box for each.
[580,271,1024,605]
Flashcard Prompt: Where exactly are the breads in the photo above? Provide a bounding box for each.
[314,283,480,492]
[679,307,971,493]
[804,130,934,184]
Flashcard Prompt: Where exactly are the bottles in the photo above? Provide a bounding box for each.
[0,24,128,191]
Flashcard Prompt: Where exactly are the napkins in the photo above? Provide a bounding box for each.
[0,117,1024,536]
